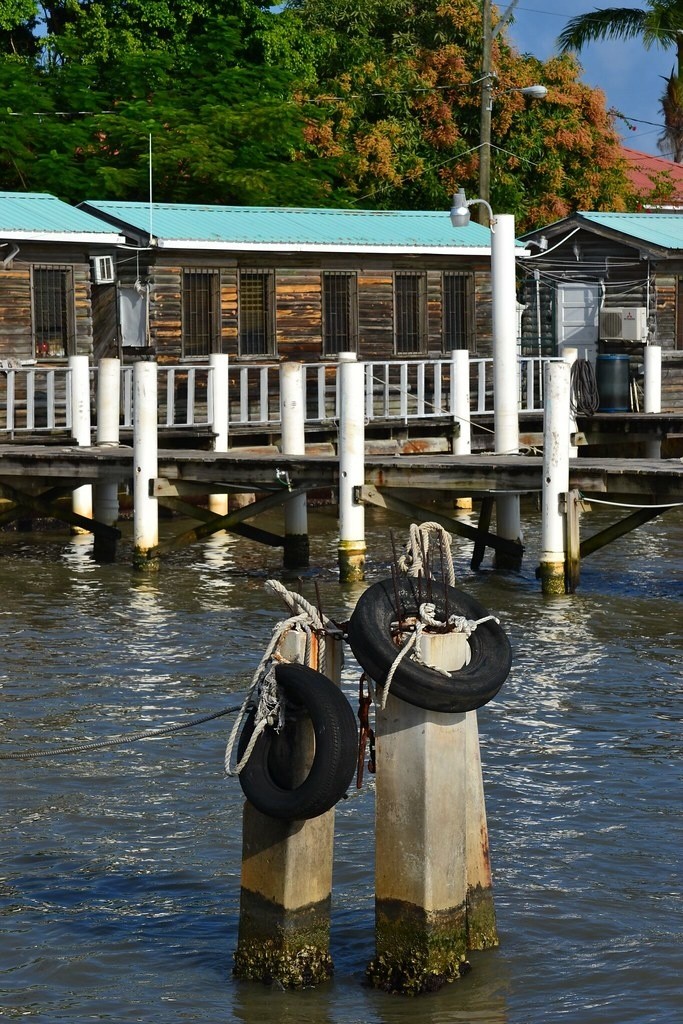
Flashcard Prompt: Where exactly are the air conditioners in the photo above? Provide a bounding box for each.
[598,306,647,342]
[88,255,116,286]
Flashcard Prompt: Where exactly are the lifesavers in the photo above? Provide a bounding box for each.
[235,661,360,821]
[347,575,514,714]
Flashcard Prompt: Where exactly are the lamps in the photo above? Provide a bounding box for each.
[447,187,498,234]
[524,235,549,253]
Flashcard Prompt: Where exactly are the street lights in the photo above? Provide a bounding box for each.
[479,84,549,228]
[453,188,522,573]
